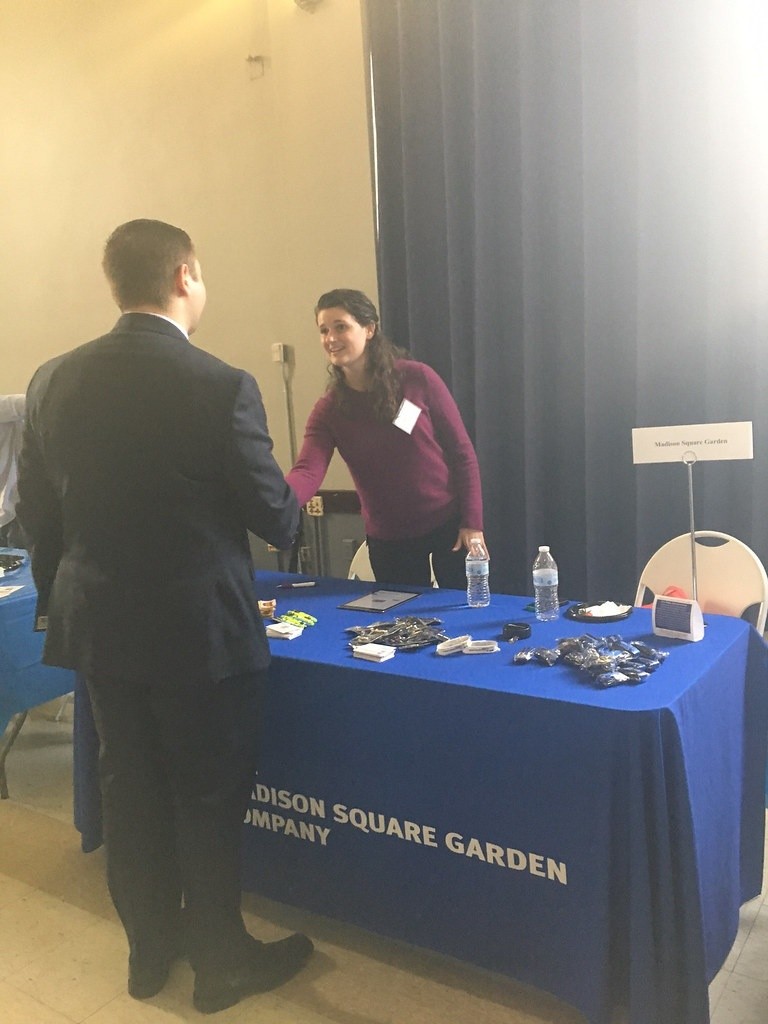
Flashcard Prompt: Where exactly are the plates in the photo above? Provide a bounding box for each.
[567,601,634,623]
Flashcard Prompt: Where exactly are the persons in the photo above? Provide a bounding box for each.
[16,219,314,1014]
[285,289,491,591]
[0,394,26,549]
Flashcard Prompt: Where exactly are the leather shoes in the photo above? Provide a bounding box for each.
[193,934,314,1014]
[127,910,189,998]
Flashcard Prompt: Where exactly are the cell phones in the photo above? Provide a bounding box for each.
[527,598,569,614]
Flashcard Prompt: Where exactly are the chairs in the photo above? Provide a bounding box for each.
[634,530,768,638]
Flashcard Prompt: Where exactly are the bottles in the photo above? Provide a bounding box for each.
[532,546,559,621]
[465,538,490,608]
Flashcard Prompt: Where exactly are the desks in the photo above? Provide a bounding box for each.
[72,569,768,1024]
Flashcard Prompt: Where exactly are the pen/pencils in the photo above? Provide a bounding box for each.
[276,581,316,588]
